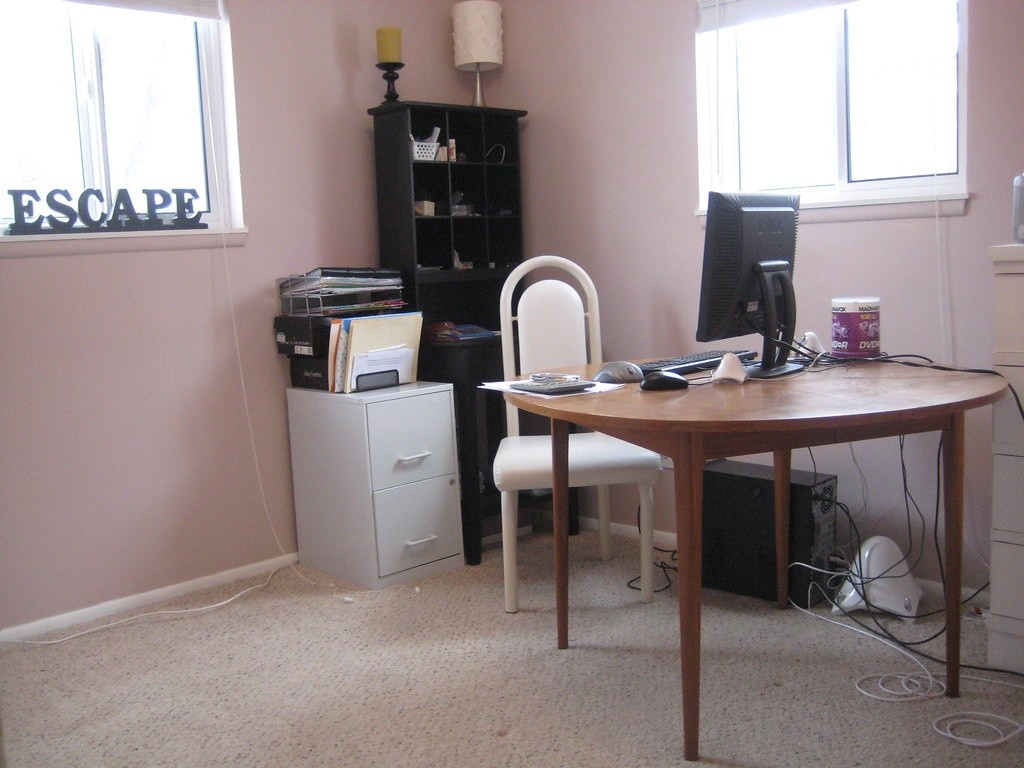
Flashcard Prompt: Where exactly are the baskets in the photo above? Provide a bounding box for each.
[411,141,440,160]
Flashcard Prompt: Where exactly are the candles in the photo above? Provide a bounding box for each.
[376,26,403,63]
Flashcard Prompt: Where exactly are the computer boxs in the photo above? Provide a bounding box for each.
[702,458,837,608]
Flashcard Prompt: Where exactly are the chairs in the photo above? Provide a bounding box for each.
[492,255,663,615]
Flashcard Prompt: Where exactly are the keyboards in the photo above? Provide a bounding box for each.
[638,348,756,376]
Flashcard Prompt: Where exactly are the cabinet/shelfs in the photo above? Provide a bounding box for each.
[285,380,466,591]
[982,244,1024,676]
[366,101,530,537]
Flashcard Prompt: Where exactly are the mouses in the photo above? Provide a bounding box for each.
[640,371,689,391]
[593,361,644,384]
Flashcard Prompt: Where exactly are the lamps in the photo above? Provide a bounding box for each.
[451,0,505,107]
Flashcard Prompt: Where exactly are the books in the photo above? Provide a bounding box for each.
[279,265,422,394]
[452,323,495,340]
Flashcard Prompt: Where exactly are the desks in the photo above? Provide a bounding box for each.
[427,334,580,566]
[503,352,1007,760]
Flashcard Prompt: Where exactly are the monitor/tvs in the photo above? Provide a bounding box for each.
[696,190,805,378]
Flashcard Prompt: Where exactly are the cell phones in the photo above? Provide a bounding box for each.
[530,373,580,382]
